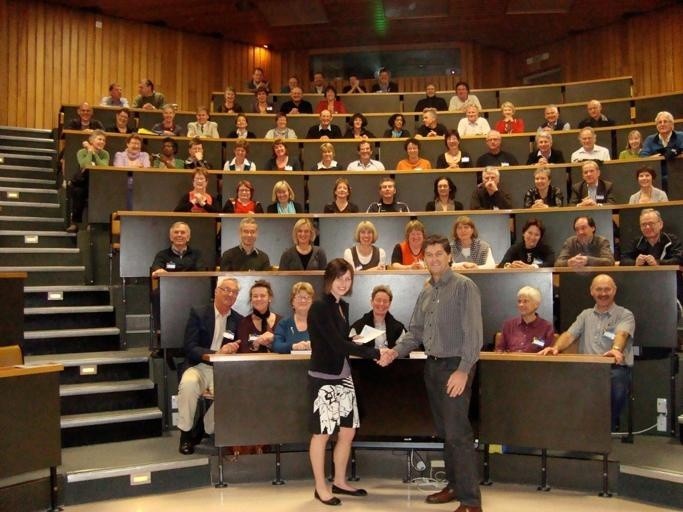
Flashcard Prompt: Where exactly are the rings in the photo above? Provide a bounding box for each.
[458,387,462,391]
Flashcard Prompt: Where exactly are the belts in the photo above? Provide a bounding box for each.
[428,354,462,360]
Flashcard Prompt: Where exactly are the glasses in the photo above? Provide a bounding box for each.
[218,286,239,295]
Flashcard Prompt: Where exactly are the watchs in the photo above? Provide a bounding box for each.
[614,345,623,353]
[166,160,171,166]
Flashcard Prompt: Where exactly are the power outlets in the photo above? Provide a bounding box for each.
[657,398,667,414]
[657,414,668,432]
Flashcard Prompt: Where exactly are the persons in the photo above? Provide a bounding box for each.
[185,138,213,169]
[177,278,244,454]
[265,112,297,138]
[344,112,374,138]
[639,112,683,157]
[280,219,327,269]
[113,133,150,168]
[629,166,668,204]
[523,167,564,208]
[324,178,358,213]
[132,79,165,110]
[383,114,410,138]
[471,166,512,210]
[450,217,496,269]
[316,86,347,114]
[619,130,642,159]
[539,106,571,131]
[281,87,313,114]
[150,223,206,357]
[539,274,635,432]
[457,103,490,136]
[378,235,482,512]
[344,221,386,271]
[280,73,303,94]
[497,218,555,269]
[311,142,342,171]
[67,130,109,232]
[151,137,184,169]
[224,138,257,171]
[308,71,334,94]
[496,102,525,134]
[186,107,219,138]
[250,87,276,114]
[527,132,565,164]
[347,141,385,171]
[238,280,285,352]
[100,83,129,108]
[174,168,217,213]
[578,100,608,127]
[105,108,136,134]
[392,221,427,269]
[306,109,341,139]
[151,104,182,137]
[265,140,301,170]
[227,114,256,139]
[396,138,432,170]
[243,67,271,94]
[554,216,614,267]
[448,81,482,111]
[223,180,263,214]
[436,129,473,169]
[372,68,398,93]
[306,259,393,506]
[571,126,610,163]
[502,287,553,353]
[217,86,243,113]
[343,74,366,94]
[414,107,448,137]
[220,218,271,271]
[425,176,464,211]
[619,208,683,266]
[273,283,314,353]
[350,285,406,349]
[414,83,448,112]
[476,130,518,167]
[267,181,302,213]
[64,102,105,132]
[568,161,616,207]
[366,179,410,213]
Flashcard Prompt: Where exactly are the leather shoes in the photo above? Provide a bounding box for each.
[315,488,340,505]
[455,504,482,512]
[427,487,458,503]
[179,437,192,453]
[332,484,367,496]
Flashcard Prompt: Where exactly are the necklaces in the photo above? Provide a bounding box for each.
[299,247,310,251]
